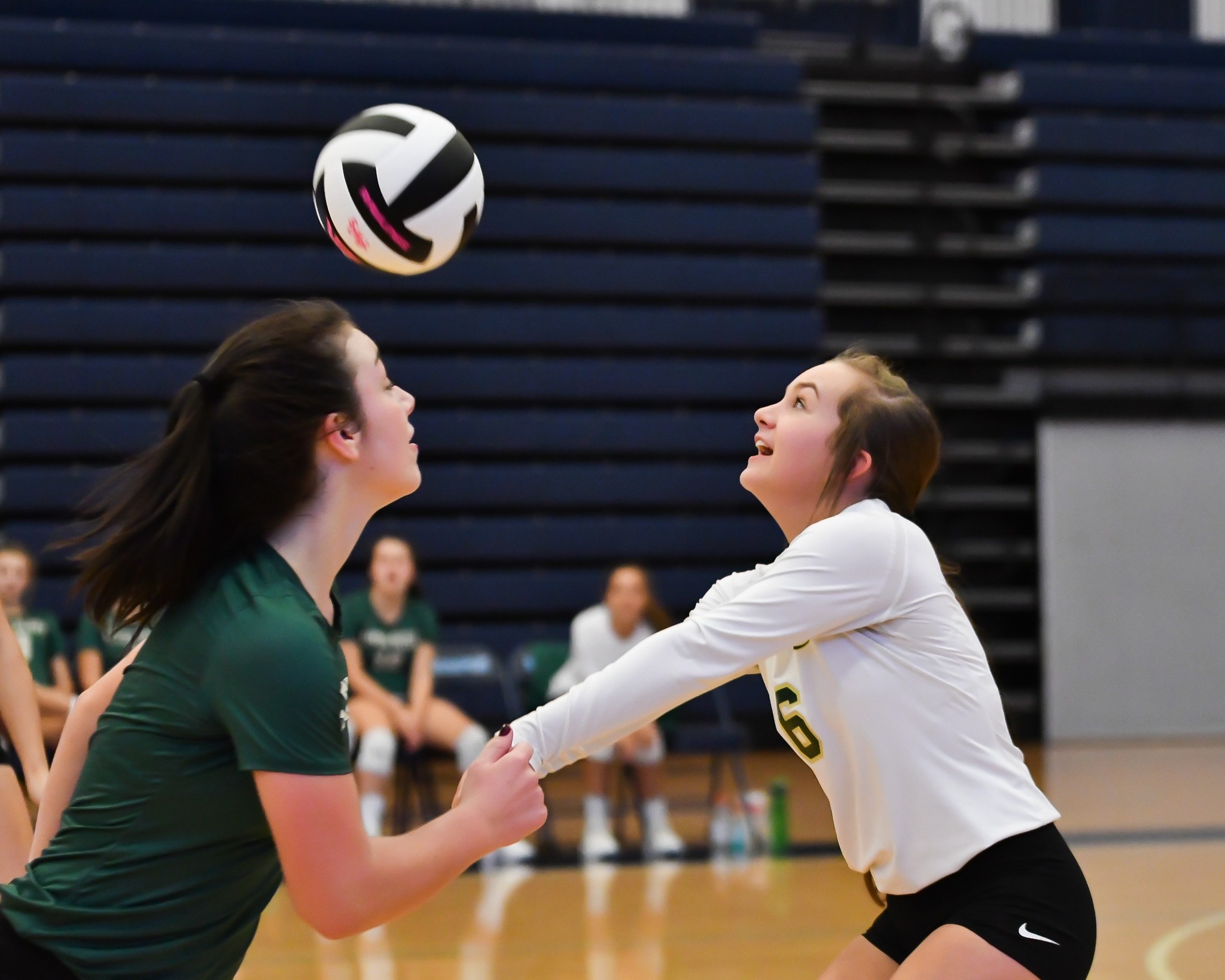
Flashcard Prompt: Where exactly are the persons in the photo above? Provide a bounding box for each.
[0,532,538,882]
[451,347,1097,980]
[0,296,549,979]
[545,564,687,863]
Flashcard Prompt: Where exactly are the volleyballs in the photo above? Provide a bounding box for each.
[313,103,485,276]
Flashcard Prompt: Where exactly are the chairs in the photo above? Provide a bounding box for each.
[389,640,764,874]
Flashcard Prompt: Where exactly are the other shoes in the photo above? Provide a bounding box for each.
[578,829,619,859]
[641,828,684,857]
[481,836,537,859]
